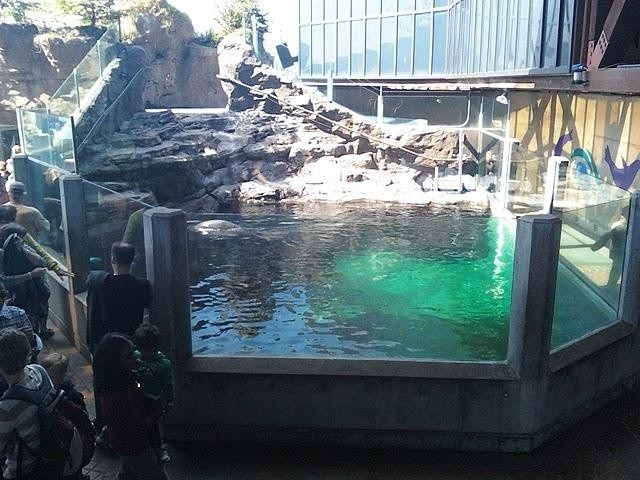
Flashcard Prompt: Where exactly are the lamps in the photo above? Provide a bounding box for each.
[495,88,509,105]
[570,62,591,88]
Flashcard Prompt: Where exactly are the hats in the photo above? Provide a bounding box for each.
[8,181,26,194]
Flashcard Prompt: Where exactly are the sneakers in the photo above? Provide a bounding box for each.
[90,418,115,451]
[156,442,172,466]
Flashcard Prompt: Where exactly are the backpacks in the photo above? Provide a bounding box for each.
[0,365,96,480]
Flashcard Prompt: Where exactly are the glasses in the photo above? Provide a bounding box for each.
[0,289,9,299]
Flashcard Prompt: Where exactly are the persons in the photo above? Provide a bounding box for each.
[591,205,629,296]
